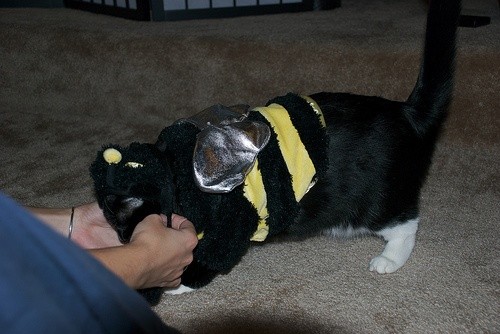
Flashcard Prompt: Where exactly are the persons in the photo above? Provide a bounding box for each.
[27,200,200,290]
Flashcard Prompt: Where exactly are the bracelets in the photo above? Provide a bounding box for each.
[66,207,77,242]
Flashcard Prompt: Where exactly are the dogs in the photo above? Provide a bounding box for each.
[88,0,461,309]
